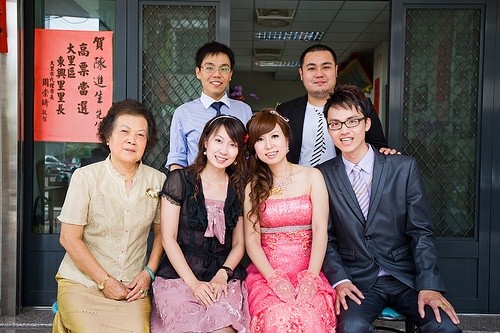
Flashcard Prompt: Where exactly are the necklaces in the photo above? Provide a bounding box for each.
[269,161,295,196]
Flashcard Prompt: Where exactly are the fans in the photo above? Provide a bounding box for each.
[253,49,282,61]
[256,8,296,27]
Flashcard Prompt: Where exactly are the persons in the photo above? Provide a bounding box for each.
[229,107,338,333]
[150,114,249,333]
[276,43,402,167]
[315,85,461,333]
[51,98,164,333]
[165,41,252,172]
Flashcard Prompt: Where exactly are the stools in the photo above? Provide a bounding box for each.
[375,307,415,333]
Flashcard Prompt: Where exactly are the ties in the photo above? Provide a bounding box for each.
[310,109,326,166]
[351,166,369,220]
[211,101,224,116]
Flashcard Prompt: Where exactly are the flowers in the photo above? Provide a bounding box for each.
[230,85,259,103]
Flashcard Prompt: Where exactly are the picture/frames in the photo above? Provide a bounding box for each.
[336,52,373,93]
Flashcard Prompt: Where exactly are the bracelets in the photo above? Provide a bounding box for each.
[143,266,155,280]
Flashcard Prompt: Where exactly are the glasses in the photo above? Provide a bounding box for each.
[199,65,230,73]
[327,116,367,130]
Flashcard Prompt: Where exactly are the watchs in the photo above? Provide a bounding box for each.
[98,274,110,292]
[219,265,234,282]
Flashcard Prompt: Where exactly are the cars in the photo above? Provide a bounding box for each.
[45,155,66,177]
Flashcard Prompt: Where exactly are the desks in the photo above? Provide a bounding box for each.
[45,185,64,234]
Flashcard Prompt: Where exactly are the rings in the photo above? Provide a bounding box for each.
[139,290,147,296]
[441,303,447,310]
[284,287,288,290]
[303,288,309,292]
